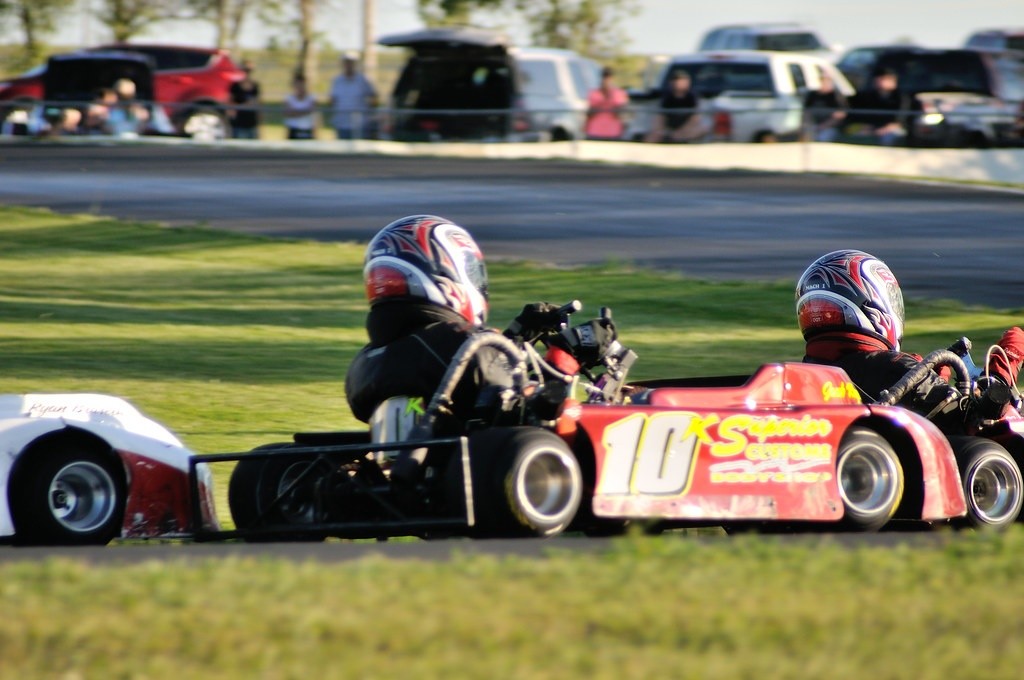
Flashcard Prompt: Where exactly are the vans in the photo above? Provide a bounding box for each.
[375,33,600,145]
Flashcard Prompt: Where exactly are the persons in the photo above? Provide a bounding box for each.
[281,72,321,140]
[584,68,718,141]
[225,62,263,140]
[794,248,1024,436]
[344,213,611,439]
[52,77,153,137]
[801,66,923,142]
[325,60,381,140]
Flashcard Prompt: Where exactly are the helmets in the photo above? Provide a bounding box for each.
[794,248,905,352]
[363,215,489,337]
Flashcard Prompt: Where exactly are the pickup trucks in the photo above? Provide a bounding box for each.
[618,51,858,144]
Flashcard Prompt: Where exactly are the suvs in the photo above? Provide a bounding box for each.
[0,41,242,140]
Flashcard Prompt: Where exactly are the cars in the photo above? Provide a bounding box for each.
[695,23,842,52]
[26,51,174,136]
[833,29,1024,149]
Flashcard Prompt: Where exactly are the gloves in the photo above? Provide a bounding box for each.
[572,317,617,347]
[984,326,1024,387]
[502,303,568,343]
[912,353,951,383]
